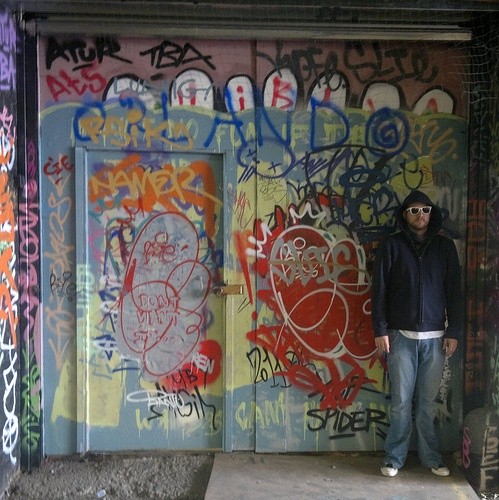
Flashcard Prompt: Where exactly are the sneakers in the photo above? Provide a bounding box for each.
[381,465,398,477]
[431,464,449,476]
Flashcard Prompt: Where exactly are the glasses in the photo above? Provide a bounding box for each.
[404,206,431,215]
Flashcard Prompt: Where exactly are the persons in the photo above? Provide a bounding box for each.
[371,188,468,478]
